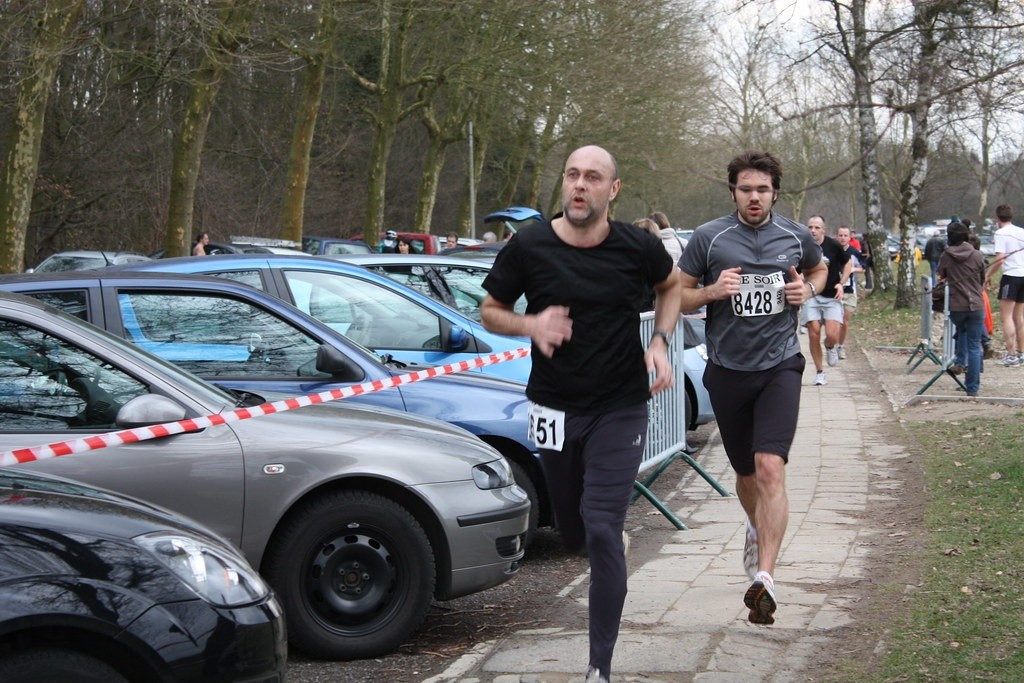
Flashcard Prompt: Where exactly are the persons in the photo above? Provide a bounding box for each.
[850,231,872,289]
[679,150,828,626]
[925,230,945,287]
[484,232,496,242]
[835,225,867,359]
[503,231,513,242]
[937,219,994,396]
[481,146,682,683]
[192,233,209,257]
[632,211,688,261]
[800,215,852,385]
[985,204,1024,367]
[437,233,461,255]
[379,230,416,255]
[895,243,923,269]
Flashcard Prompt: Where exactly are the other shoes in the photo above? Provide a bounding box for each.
[800,326,806,334]
[584,665,609,683]
[950,365,964,375]
[622,529,630,557]
[957,388,965,390]
[983,343,993,359]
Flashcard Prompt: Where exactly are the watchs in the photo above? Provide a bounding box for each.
[650,329,671,345]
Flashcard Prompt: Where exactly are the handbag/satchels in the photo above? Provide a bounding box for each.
[931,281,944,312]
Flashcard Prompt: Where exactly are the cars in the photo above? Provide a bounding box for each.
[0,290,532,683]
[853,219,997,257]
[0,206,715,554]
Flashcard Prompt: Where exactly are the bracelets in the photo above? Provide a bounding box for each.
[839,282,843,285]
[808,280,817,296]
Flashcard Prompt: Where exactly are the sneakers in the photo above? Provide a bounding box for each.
[814,373,827,385]
[744,574,777,624]
[824,337,837,367]
[743,516,758,580]
[837,344,845,360]
[1017,353,1024,365]
[996,354,1019,367]
